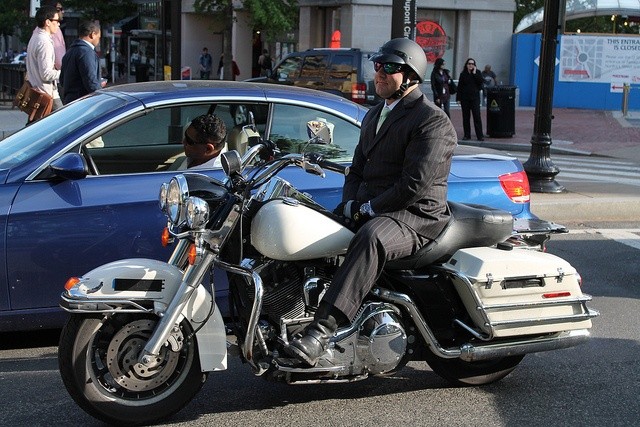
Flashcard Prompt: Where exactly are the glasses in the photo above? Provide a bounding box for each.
[49,19,61,22]
[57,7,64,11]
[373,61,408,74]
[467,63,475,65]
[185,129,221,145]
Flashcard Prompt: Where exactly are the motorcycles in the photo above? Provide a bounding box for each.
[56,110,601,427]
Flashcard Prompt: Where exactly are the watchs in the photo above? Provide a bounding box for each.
[359,201,373,219]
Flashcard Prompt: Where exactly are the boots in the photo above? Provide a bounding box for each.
[283,299,346,366]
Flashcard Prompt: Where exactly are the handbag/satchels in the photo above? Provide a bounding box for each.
[447,71,457,94]
[11,79,52,120]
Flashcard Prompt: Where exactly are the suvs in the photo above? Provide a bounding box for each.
[228,46,382,129]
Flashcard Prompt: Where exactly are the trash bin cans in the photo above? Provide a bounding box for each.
[488,85,518,138]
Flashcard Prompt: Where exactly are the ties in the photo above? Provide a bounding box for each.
[375,106,390,133]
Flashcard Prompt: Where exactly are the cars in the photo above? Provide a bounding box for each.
[0,80,533,338]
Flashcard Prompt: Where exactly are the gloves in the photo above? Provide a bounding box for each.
[333,199,373,223]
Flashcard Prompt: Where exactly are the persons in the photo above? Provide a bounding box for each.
[258,49,275,77]
[26,6,61,126]
[117,50,124,77]
[50,3,65,84]
[217,56,225,79]
[199,47,211,80]
[167,115,228,173]
[431,57,456,119]
[231,56,239,80]
[286,38,458,367]
[58,17,108,105]
[482,65,497,103]
[457,58,484,141]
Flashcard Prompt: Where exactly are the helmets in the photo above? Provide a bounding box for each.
[368,37,427,99]
